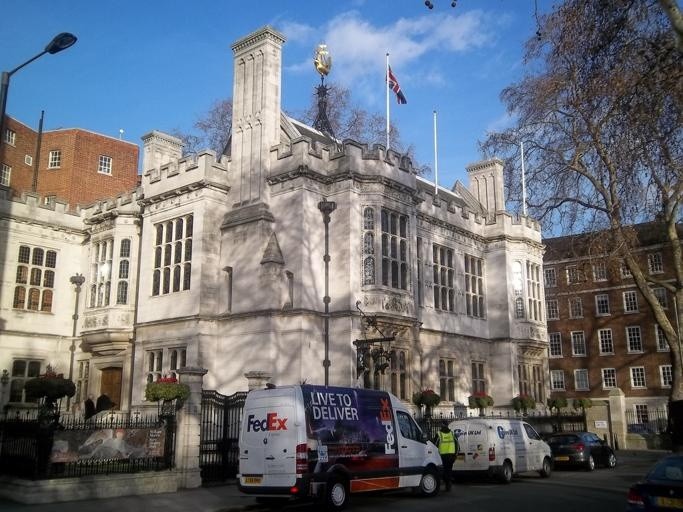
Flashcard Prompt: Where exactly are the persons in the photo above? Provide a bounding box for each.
[432,419,459,492]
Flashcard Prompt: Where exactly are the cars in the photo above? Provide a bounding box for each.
[547,431,617,471]
[627,454,683,512]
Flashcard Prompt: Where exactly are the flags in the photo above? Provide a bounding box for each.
[384,63,407,106]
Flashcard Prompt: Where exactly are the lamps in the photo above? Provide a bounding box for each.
[353,337,394,376]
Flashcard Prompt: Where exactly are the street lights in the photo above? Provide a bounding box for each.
[0,33,77,139]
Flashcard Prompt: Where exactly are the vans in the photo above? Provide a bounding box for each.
[238,383,444,512]
[447,418,553,484]
